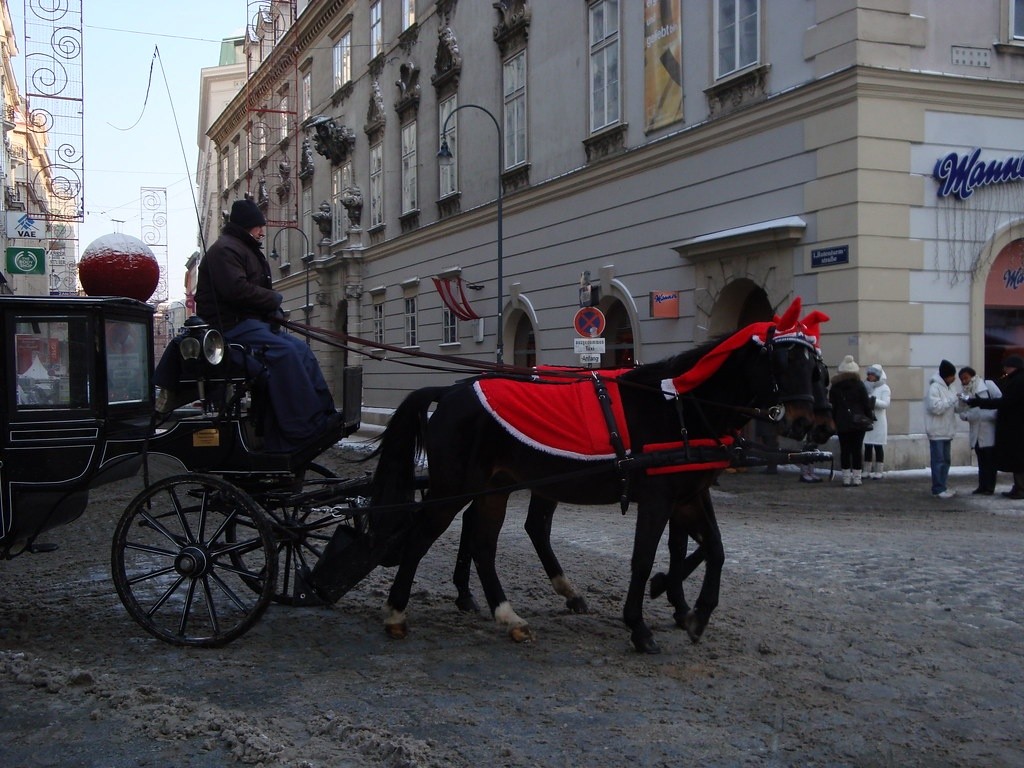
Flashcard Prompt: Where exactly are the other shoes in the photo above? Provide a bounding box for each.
[1001,485,1024,499]
[972,486,994,495]
[933,489,957,499]
[293,408,345,451]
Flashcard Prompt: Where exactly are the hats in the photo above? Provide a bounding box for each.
[867,364,883,378]
[1003,356,1024,368]
[230,199,266,229]
[838,355,859,373]
[940,360,956,378]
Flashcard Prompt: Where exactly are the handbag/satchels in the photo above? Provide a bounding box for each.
[849,413,874,431]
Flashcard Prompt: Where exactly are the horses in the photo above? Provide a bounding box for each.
[368,296,839,658]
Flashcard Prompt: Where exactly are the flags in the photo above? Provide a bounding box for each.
[431,277,480,321]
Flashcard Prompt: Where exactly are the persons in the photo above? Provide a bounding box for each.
[155,316,206,403]
[924,359,958,499]
[966,356,1024,500]
[193,200,344,448]
[828,354,876,486]
[861,364,891,479]
[957,367,1002,496]
[799,442,823,483]
[759,432,779,474]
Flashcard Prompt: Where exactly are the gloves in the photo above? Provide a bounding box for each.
[269,308,285,329]
[966,393,982,408]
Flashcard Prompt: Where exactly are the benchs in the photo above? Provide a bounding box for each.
[155,333,267,432]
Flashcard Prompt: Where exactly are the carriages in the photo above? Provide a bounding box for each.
[0,293,836,652]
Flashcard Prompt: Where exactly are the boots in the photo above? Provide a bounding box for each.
[841,468,863,486]
[861,461,884,479]
[799,463,823,483]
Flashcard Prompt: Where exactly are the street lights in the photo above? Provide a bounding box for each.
[437,105,505,369]
[270,227,311,350]
